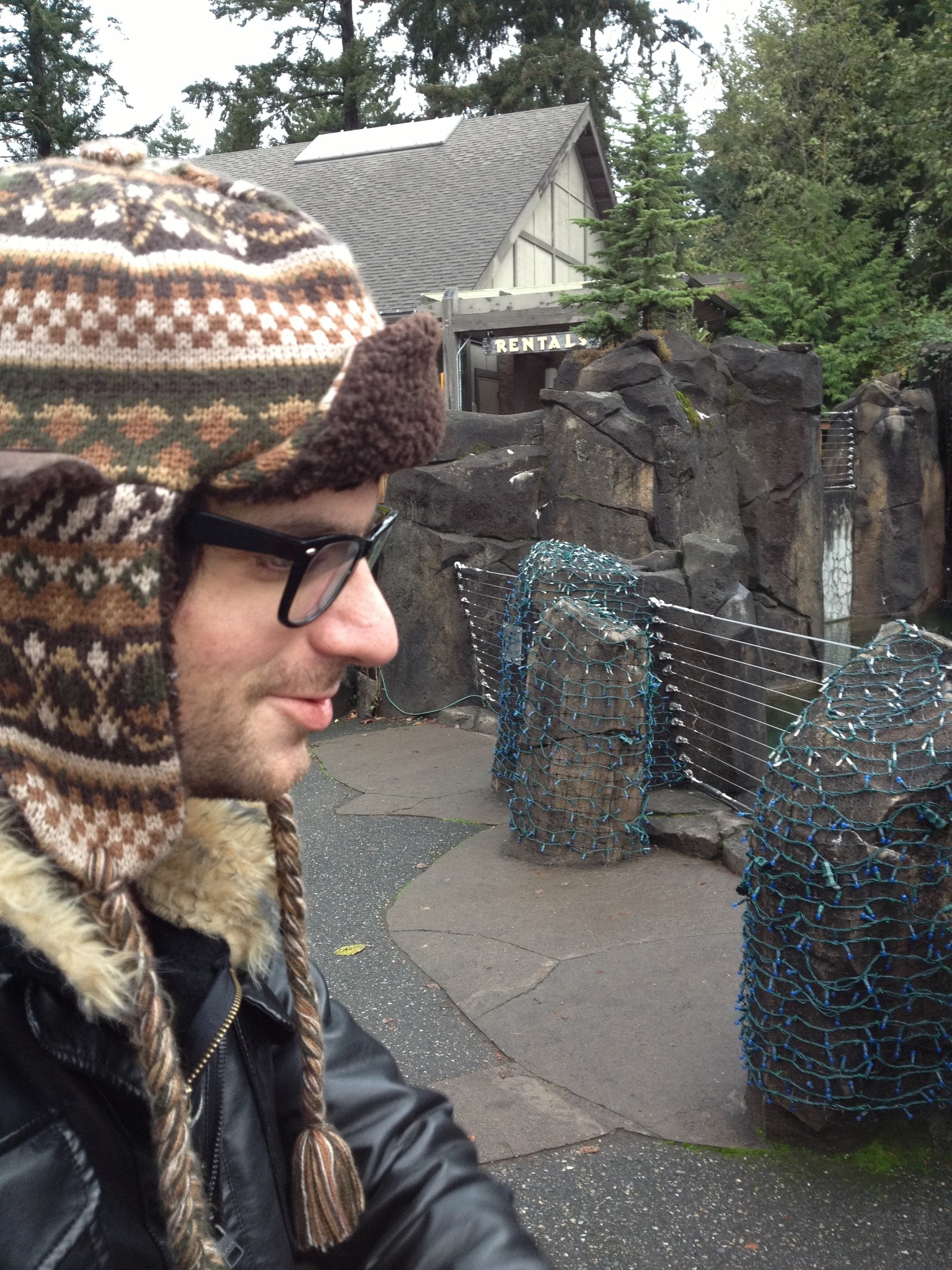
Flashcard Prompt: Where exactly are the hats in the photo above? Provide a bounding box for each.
[0,136,454,1269]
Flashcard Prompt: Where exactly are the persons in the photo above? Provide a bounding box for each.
[0,140,556,1270]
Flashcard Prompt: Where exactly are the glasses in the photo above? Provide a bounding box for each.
[176,500,400,628]
